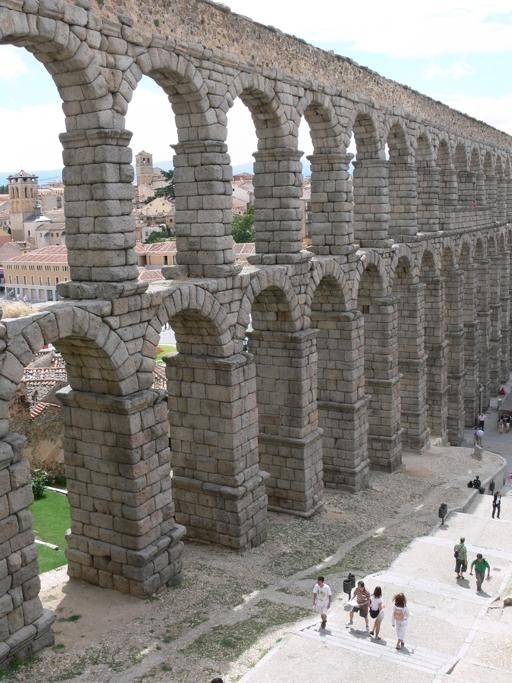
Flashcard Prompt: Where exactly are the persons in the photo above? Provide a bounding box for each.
[312,575,333,629]
[489,478,496,494]
[452,536,469,580]
[472,383,511,446]
[470,551,490,594]
[368,586,386,640]
[492,490,501,518]
[473,475,482,488]
[345,580,371,631]
[391,591,410,649]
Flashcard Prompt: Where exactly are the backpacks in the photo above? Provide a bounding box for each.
[395,607,404,620]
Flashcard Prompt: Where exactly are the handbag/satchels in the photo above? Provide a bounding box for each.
[370,607,379,618]
[454,551,459,558]
